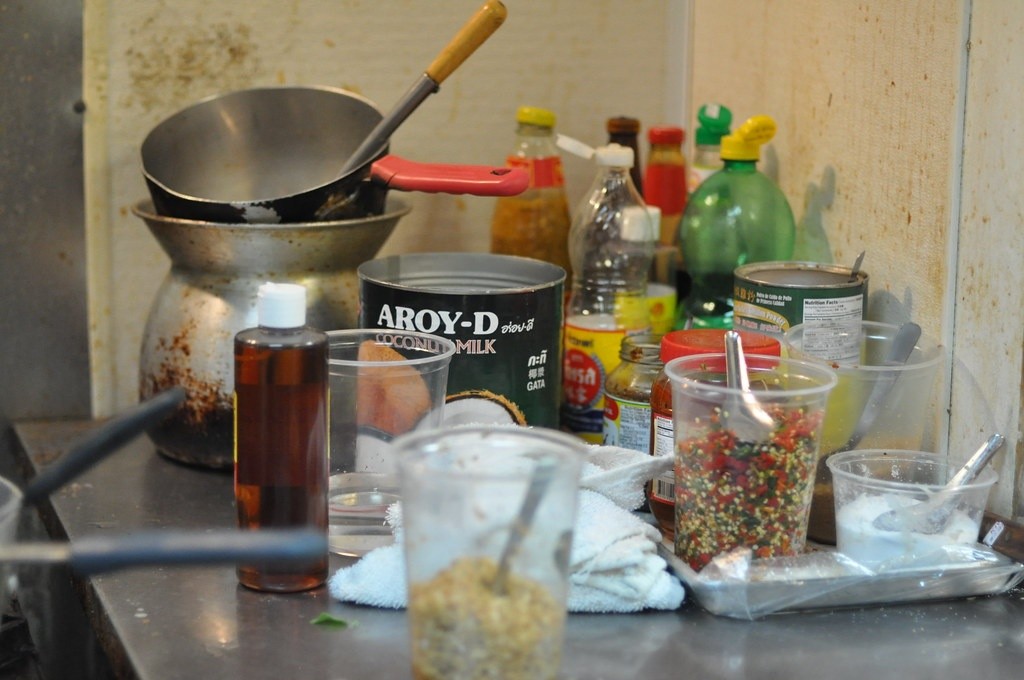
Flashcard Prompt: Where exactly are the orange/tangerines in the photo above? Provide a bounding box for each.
[356,342,427,441]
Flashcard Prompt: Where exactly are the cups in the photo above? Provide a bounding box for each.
[390,423,588,679]
[325,329,453,556]
[664,353,837,578]
[785,318,944,544]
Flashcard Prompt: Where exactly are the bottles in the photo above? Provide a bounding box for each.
[607,118,657,285]
[556,135,652,445]
[234,283,328,592]
[688,104,732,204]
[644,126,688,284]
[490,107,573,323]
[674,116,795,329]
[621,207,676,344]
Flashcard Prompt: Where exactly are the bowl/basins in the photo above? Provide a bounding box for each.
[826,449,996,570]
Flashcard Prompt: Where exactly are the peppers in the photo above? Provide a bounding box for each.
[676,410,821,572]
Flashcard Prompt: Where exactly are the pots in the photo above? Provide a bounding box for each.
[140,88,528,222]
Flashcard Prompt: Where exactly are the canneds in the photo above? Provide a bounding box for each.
[652,332,783,543]
[605,336,665,455]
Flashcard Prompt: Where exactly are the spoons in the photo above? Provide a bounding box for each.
[873,434,1004,535]
[719,330,774,444]
[816,322,922,484]
